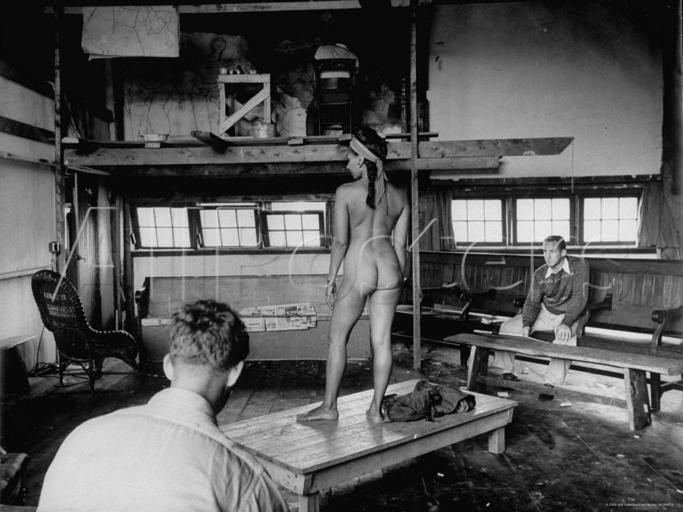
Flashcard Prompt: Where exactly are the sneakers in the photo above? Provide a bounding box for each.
[496,373,555,401]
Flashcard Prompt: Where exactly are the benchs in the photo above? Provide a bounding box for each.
[392,251,683,414]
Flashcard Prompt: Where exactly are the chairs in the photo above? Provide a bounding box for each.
[0,446,30,507]
[315,59,355,136]
[31,270,148,394]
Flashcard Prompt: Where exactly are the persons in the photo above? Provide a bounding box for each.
[293,123,411,427]
[484,234,592,405]
[35,296,292,512]
[246,19,406,142]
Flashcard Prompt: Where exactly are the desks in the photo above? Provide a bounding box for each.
[443,333,683,432]
[216,73,272,140]
[214,379,518,512]
